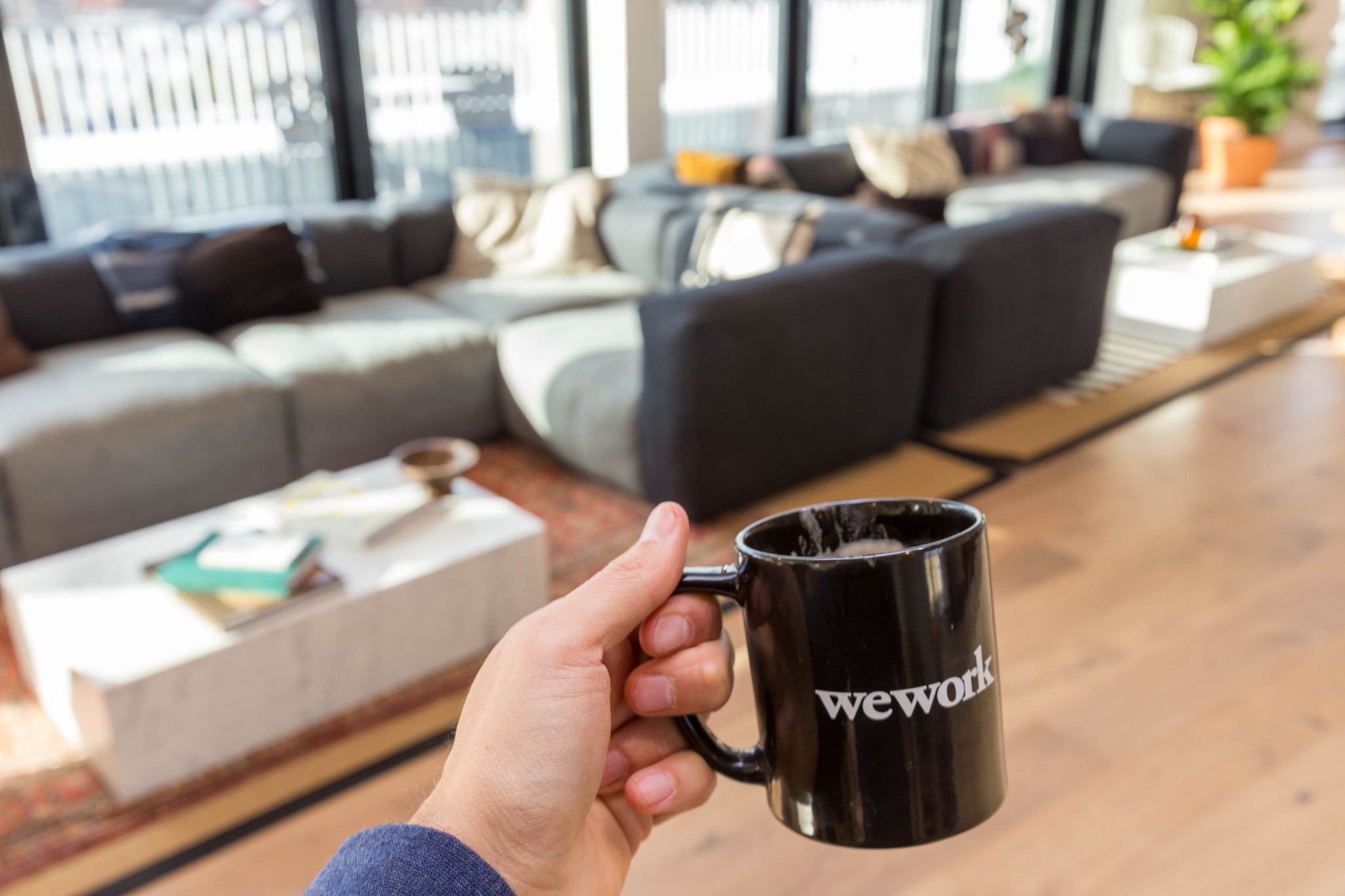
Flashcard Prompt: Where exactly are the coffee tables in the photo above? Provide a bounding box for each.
[1107,209,1326,334]
[7,458,550,811]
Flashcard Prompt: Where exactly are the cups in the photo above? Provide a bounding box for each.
[640,496,1008,849]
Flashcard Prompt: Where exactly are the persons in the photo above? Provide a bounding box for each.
[300,500,733,896]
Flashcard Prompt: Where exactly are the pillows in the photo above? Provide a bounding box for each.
[178,219,318,332]
[92,227,182,316]
[680,202,822,289]
[449,170,613,275]
[677,149,741,186]
[847,119,962,196]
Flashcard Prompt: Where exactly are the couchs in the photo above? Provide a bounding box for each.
[631,95,1192,424]
[0,178,935,527]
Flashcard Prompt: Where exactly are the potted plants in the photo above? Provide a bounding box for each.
[1192,0,1317,192]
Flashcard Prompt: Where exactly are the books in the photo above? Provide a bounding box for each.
[142,533,343,632]
